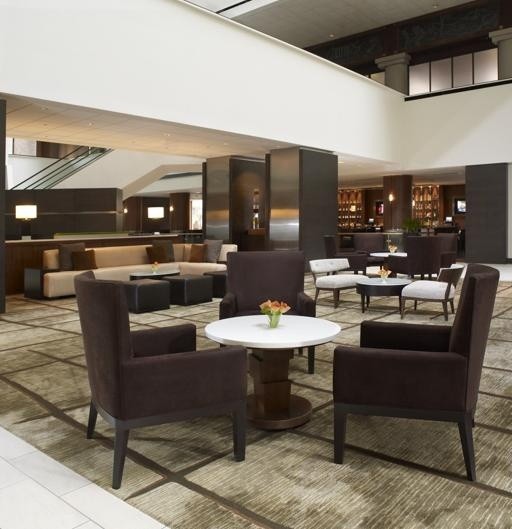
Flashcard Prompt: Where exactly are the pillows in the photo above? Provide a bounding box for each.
[57,238,224,273]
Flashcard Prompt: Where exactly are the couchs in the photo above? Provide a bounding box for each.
[25,237,240,301]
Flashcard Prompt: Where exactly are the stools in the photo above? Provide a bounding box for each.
[120,261,228,314]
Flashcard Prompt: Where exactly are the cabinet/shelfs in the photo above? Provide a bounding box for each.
[337,187,365,230]
[412,183,444,230]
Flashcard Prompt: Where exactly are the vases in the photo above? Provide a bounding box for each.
[264,312,283,330]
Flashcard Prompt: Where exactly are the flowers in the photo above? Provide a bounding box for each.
[258,298,292,329]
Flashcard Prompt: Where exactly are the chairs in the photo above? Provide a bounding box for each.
[330,260,501,483]
[306,226,468,326]
[73,268,251,493]
[217,249,318,376]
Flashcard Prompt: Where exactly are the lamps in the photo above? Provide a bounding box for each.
[146,206,165,235]
[15,204,39,240]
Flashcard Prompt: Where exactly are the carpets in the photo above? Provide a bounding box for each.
[2,278,511,528]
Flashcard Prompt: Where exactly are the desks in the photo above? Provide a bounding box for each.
[204,312,343,434]
